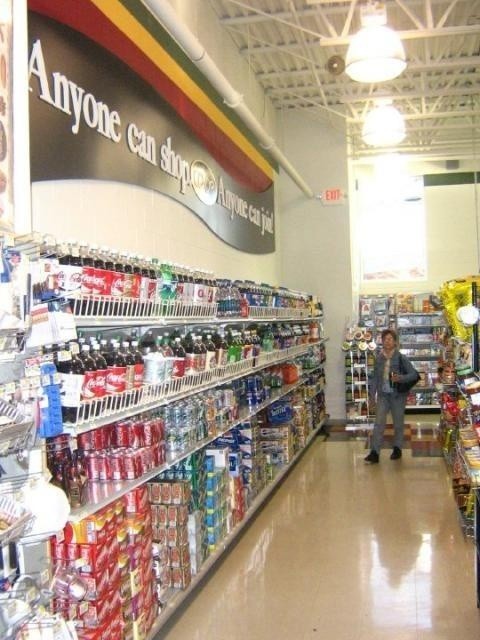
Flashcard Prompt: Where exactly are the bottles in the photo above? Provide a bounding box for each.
[390,368,396,387]
[45,240,219,316]
[219,279,322,317]
[230,320,327,384]
[40,326,229,422]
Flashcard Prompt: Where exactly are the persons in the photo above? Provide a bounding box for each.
[364,329,419,463]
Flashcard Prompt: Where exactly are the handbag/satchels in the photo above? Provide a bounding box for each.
[397,369,420,393]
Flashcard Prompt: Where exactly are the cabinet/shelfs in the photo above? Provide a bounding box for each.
[345,313,446,413]
[2,309,333,639]
[439,336,478,540]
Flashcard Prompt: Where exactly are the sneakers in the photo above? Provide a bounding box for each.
[365,450,379,463]
[390,446,401,459]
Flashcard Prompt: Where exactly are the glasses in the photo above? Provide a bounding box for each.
[383,337,393,342]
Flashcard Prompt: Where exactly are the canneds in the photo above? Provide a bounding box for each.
[81,370,284,482]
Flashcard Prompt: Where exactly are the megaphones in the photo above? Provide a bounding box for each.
[324,55,346,76]
